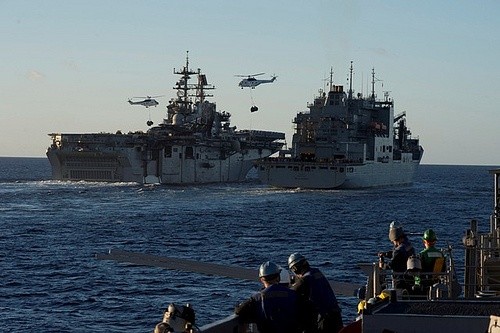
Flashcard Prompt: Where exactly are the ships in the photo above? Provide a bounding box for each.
[256,60,424,191]
[45,49,287,187]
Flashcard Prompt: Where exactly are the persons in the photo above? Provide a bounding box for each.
[128,131,141,135]
[288,252,343,333]
[234,261,314,333]
[378,221,417,295]
[116,130,121,135]
[263,157,353,163]
[416,228,447,287]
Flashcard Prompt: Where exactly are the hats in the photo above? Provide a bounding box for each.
[389,228,404,242]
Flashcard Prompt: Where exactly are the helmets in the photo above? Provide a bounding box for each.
[258,261,282,277]
[423,229,438,242]
[288,252,306,268]
[390,220,402,228]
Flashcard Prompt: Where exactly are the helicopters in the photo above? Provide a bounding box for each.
[127,95,164,108]
[233,72,279,90]
